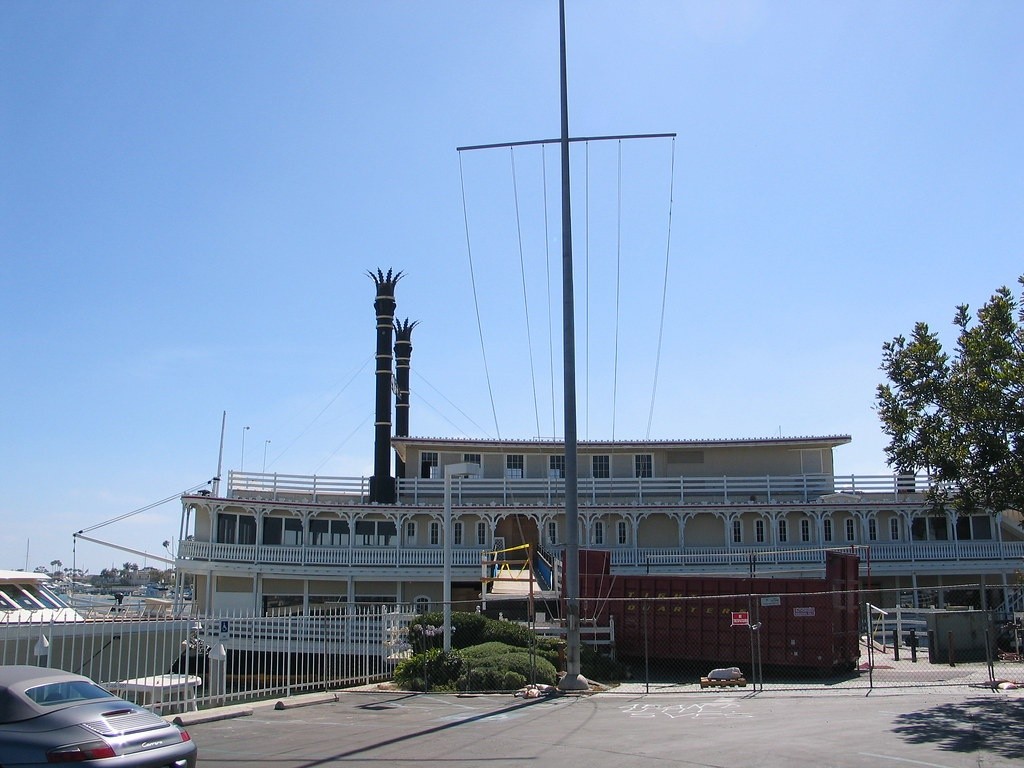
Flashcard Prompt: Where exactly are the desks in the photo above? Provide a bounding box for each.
[100,674,202,711]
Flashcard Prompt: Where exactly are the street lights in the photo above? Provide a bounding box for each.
[242,426,251,472]
[262,439,272,472]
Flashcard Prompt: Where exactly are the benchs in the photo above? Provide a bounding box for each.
[700,677,747,688]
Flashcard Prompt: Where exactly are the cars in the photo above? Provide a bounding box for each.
[0,665,199,768]
